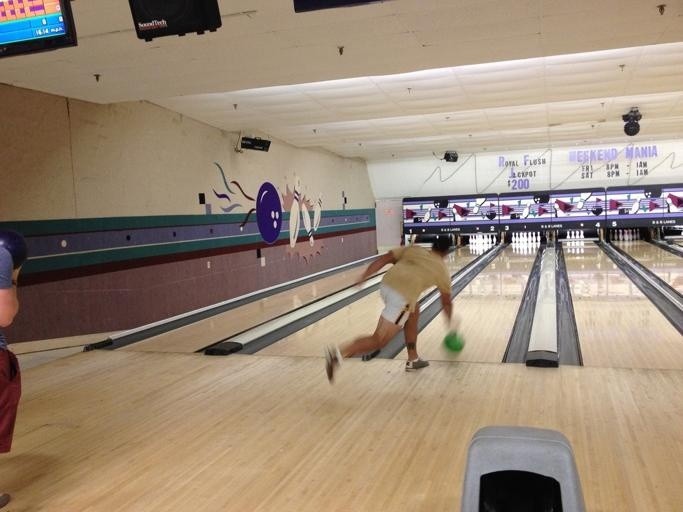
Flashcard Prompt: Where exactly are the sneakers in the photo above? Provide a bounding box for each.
[326,345,339,380]
[406,358,428,371]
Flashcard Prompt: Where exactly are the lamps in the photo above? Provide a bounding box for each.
[622,109,642,136]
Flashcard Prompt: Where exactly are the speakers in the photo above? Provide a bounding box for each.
[242,137,272,154]
[444,152,459,162]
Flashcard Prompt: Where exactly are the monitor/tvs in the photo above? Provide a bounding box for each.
[0,0,78,58]
[127,0,223,44]
[293,0,382,14]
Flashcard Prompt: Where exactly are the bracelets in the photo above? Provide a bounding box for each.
[12,280,16,285]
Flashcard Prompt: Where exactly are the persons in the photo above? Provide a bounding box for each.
[0,246,22,508]
[326,235,452,380]
